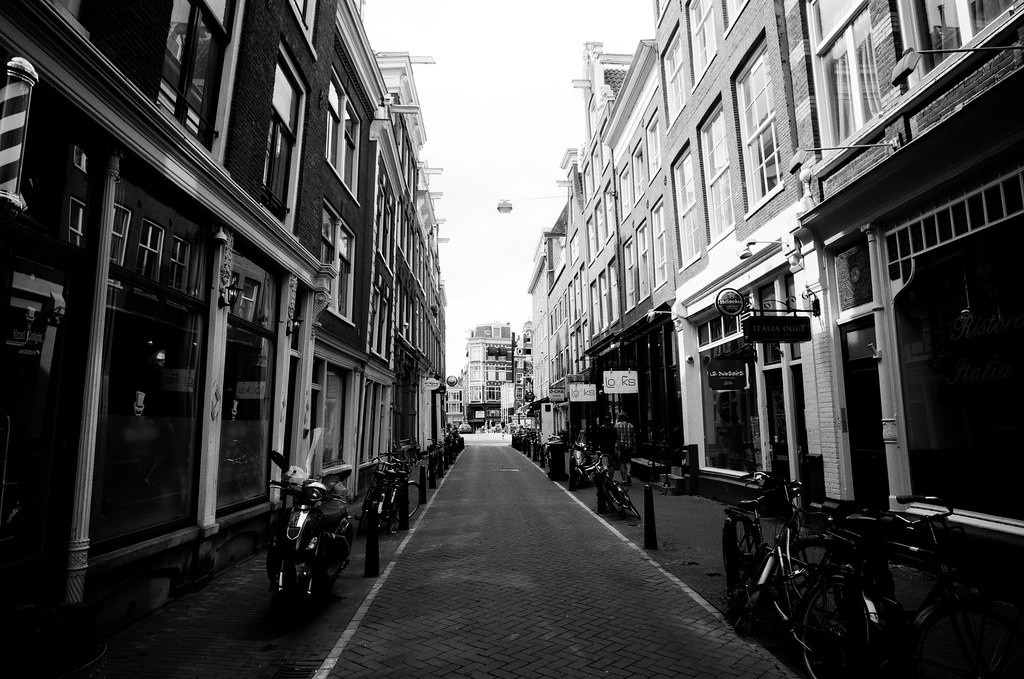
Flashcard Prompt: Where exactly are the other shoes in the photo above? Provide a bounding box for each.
[622,481,627,486]
[626,473,632,486]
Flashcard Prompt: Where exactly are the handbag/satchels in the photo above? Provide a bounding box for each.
[613,440,624,460]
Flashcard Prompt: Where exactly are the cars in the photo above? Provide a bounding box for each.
[458,424,472,434]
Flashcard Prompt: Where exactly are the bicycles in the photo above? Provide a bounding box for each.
[584,454,642,521]
[722,471,1024,679]
[427,438,444,479]
[354,451,422,537]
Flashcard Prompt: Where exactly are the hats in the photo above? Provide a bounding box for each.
[603,415,612,420]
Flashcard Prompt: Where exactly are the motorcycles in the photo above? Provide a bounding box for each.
[266,427,354,623]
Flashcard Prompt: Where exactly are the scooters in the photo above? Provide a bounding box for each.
[569,440,592,489]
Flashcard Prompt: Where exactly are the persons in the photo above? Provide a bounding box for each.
[501,419,505,433]
[599,416,620,481]
[727,415,743,467]
[487,419,490,433]
[513,420,516,433]
[589,419,599,455]
[491,419,495,433]
[615,414,636,486]
